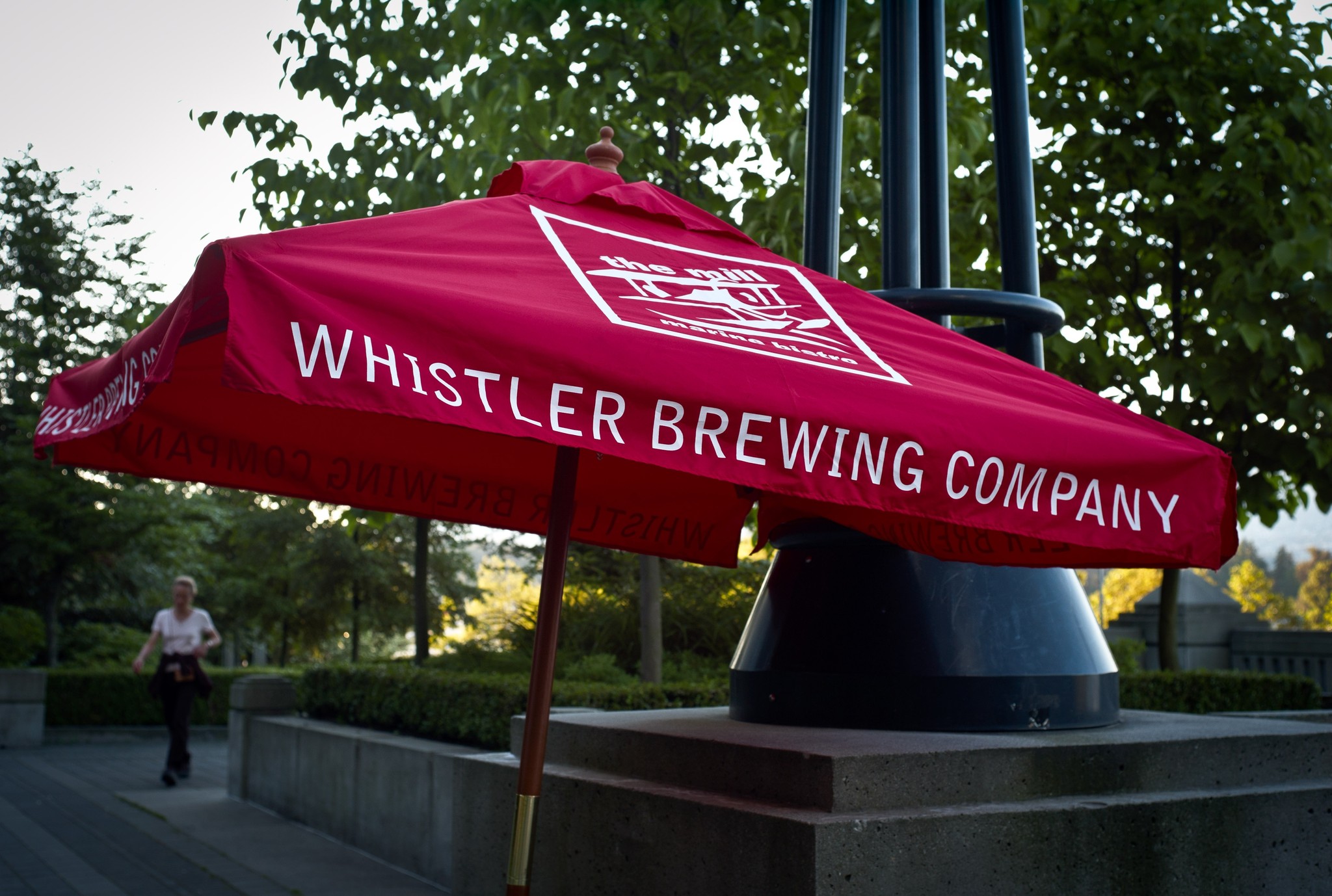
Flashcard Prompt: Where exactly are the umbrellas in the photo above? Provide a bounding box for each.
[33,124,1239,896]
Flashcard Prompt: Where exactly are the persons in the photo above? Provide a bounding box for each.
[134,575,223,786]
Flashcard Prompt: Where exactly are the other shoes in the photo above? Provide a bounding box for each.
[161,768,178,787]
[175,766,192,780]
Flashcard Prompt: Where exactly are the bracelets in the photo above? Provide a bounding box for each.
[207,640,213,647]
